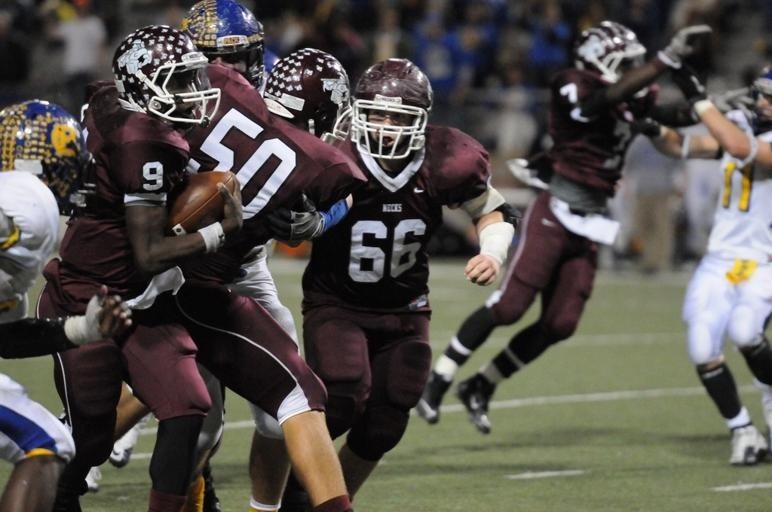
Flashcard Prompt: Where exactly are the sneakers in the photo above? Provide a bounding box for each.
[728,392,771,464]
[415,379,438,424]
[109,422,148,468]
[456,380,491,434]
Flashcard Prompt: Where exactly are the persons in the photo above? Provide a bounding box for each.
[410,16,715,435]
[372,1,771,272]
[276,56,519,512]
[2,1,371,512]
[616,68,772,466]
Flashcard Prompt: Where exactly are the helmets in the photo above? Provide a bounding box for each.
[0,99,99,218]
[565,20,647,84]
[753,64,772,95]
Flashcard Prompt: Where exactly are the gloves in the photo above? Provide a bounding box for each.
[658,24,713,110]
[623,106,660,138]
[711,86,759,120]
[262,191,324,249]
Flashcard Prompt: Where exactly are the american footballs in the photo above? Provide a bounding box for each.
[163,170,234,238]
